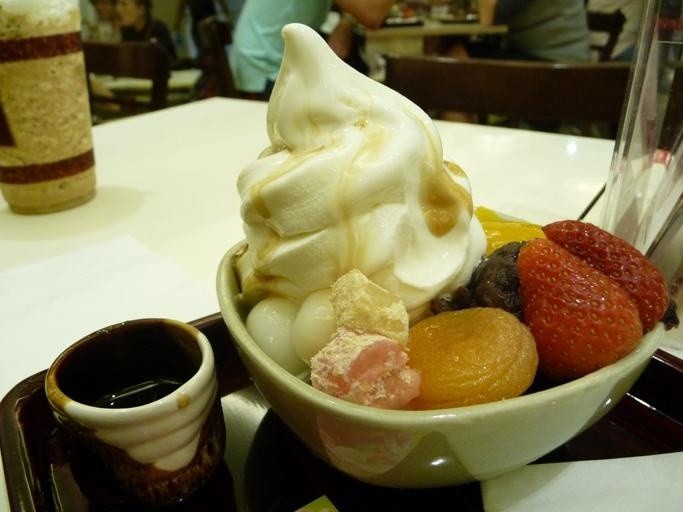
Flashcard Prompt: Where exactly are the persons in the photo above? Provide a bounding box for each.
[81,0,682,125]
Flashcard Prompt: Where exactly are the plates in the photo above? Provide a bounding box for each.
[216,204,665,485]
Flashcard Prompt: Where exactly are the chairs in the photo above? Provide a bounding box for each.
[579,10,626,136]
[82,42,169,119]
[197,18,239,98]
[381,51,633,140]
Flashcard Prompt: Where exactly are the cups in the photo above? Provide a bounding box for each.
[45,317,228,512]
[1,2,97,217]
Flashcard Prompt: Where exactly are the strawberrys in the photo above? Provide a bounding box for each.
[517,238,643,380]
[542,219,670,335]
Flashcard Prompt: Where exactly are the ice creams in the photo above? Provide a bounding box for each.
[234,22,490,386]
